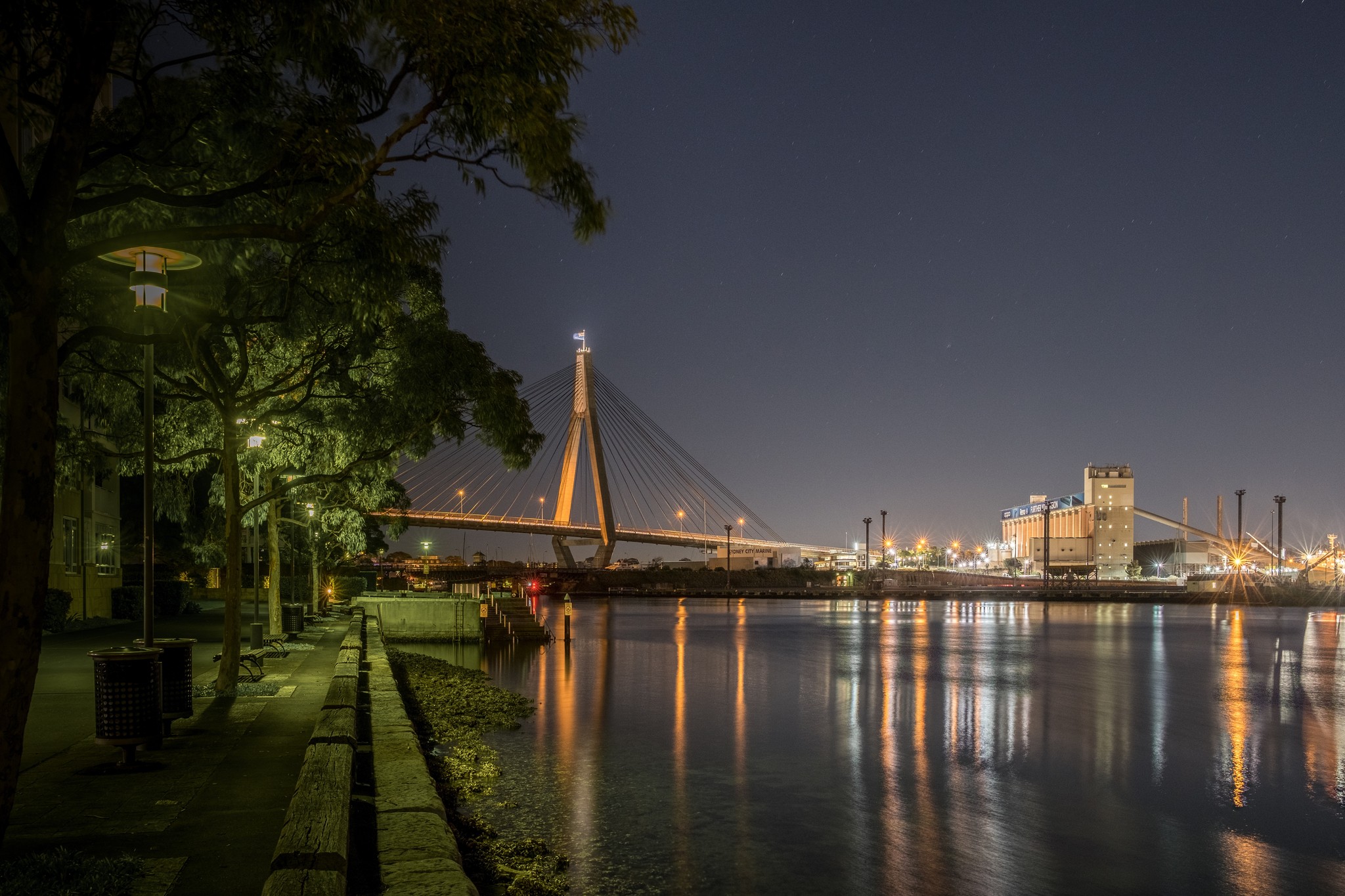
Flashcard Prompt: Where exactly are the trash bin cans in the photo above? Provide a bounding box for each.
[87,646,164,744]
[280,604,301,633]
[133,638,199,718]
[284,602,305,632]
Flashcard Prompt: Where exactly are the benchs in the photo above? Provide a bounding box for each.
[263,632,290,659]
[304,614,318,628]
[304,611,325,622]
[211,648,266,681]
